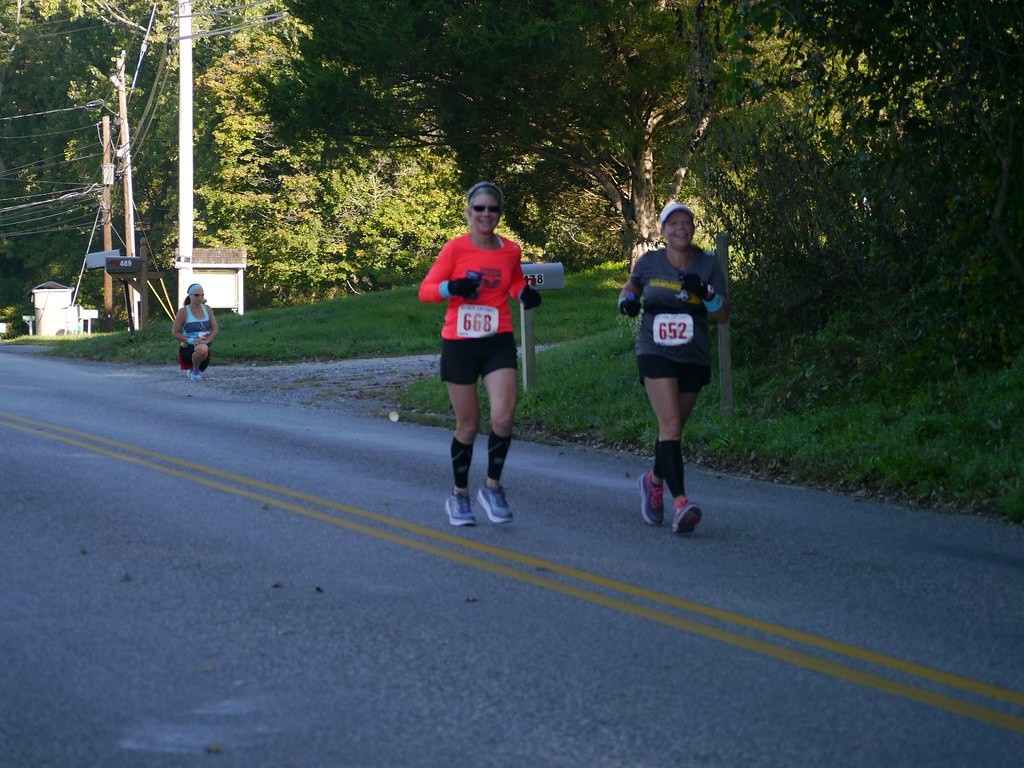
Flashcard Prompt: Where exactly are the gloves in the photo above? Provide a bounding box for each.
[448,279,479,298]
[520,285,541,309]
[620,299,641,316]
[679,271,713,301]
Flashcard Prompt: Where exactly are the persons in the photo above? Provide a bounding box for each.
[171,283,220,381]
[418,182,543,527]
[618,204,729,533]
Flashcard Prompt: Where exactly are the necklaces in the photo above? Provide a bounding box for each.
[190,304,206,330]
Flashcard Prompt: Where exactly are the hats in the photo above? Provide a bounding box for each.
[661,202,693,225]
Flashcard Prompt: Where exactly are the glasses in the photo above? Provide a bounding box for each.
[192,293,204,297]
[471,205,501,213]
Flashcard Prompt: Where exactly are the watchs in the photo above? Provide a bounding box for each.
[704,284,715,300]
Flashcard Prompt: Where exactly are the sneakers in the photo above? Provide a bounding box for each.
[638,470,665,525]
[189,370,201,380]
[476,478,513,523]
[445,486,476,526]
[672,497,702,533]
